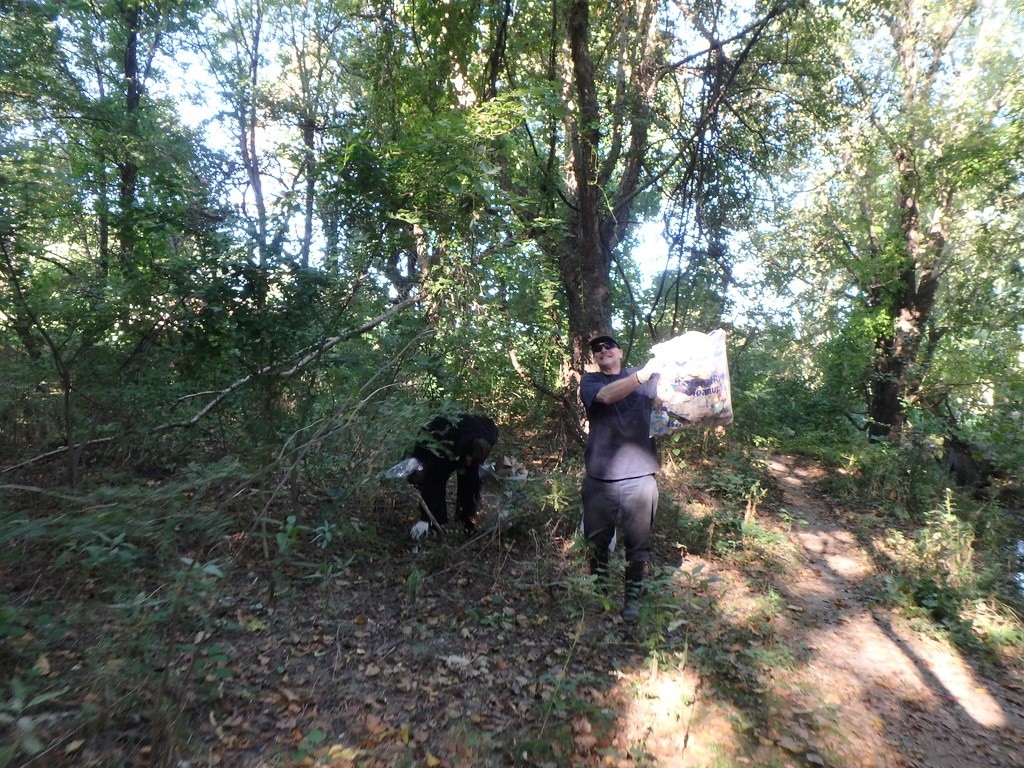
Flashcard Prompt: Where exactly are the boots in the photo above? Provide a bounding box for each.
[585,554,609,614]
[621,562,644,625]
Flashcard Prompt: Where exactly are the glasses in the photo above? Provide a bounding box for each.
[593,342,617,353]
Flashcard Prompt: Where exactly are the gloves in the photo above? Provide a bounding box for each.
[410,522,429,541]
[636,358,660,384]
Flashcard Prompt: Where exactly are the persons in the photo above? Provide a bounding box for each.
[387,402,501,538]
[579,335,662,626]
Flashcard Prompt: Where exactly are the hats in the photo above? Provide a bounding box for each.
[587,332,621,349]
